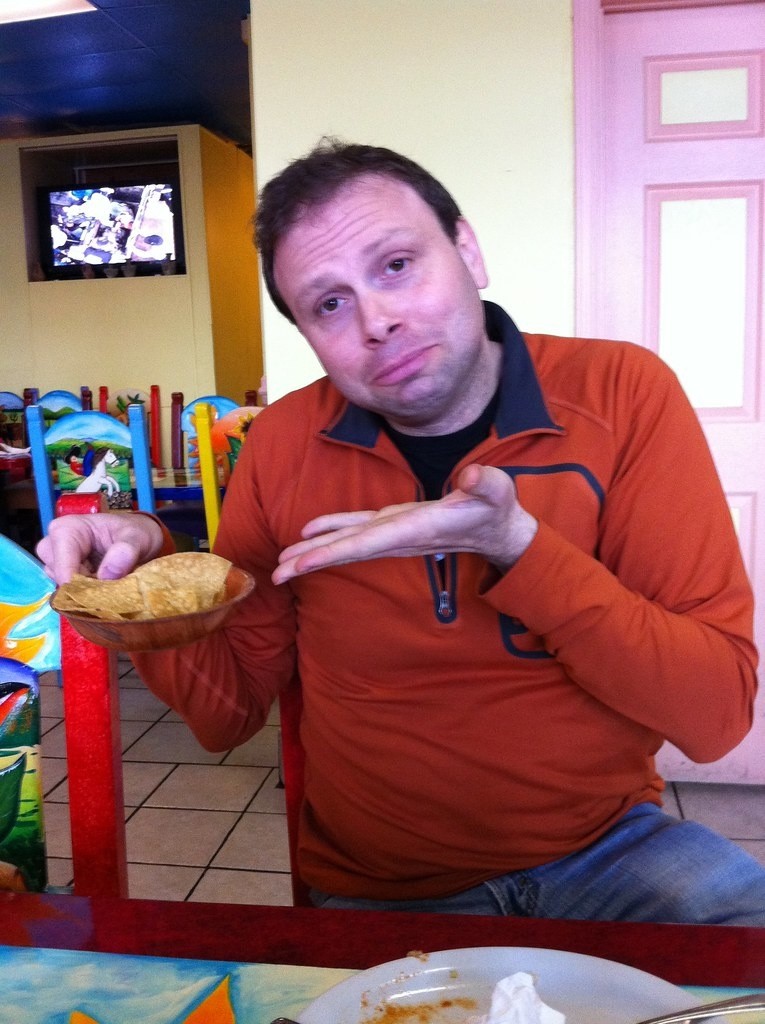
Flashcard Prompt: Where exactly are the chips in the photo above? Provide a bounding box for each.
[49,551,231,620]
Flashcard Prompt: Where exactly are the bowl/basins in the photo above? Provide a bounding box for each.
[49,565,255,651]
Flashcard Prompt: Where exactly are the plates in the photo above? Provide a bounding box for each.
[291,943,730,1024]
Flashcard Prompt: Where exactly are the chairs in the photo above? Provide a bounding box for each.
[23,386,93,434]
[24,404,158,539]
[0,391,30,449]
[171,390,258,470]
[98,385,162,468]
[0,535,134,899]
[194,401,266,554]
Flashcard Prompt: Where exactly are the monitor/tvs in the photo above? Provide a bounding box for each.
[34,175,187,280]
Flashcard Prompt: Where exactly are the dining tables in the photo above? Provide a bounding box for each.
[0,889,765,1024]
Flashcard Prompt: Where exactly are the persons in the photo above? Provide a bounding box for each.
[33,138,765,924]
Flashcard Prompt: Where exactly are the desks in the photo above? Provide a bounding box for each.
[5,465,225,512]
